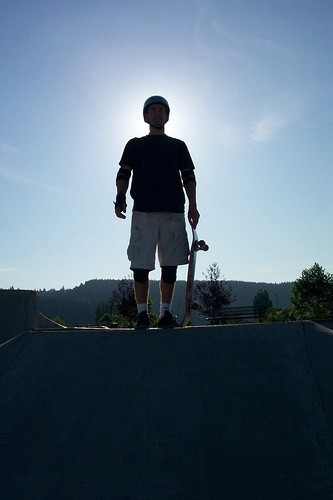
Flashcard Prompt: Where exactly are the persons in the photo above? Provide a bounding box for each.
[114,95,200,330]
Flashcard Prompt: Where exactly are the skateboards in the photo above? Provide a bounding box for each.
[180,219,208,327]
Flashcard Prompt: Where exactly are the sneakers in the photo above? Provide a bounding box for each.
[157,310,178,327]
[134,310,151,330]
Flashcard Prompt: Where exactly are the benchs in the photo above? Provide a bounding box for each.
[205,306,263,325]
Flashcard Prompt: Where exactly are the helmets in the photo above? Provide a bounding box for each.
[143,96,170,111]
